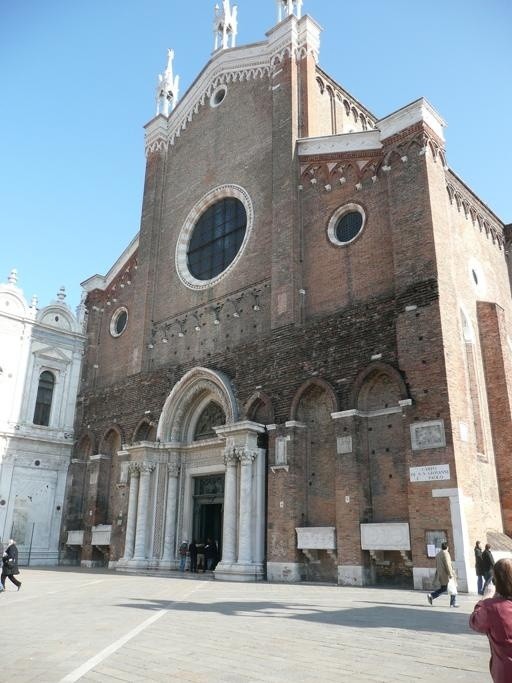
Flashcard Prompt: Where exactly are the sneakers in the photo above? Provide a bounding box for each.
[451,604,459,608]
[16,582,22,592]
[428,594,433,604]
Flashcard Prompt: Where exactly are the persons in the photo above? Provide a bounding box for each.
[426,540,460,608]
[473,540,491,595]
[480,543,498,595]
[467,557,511,683]
[179,535,218,573]
[1,538,22,591]
[0,537,4,592]
